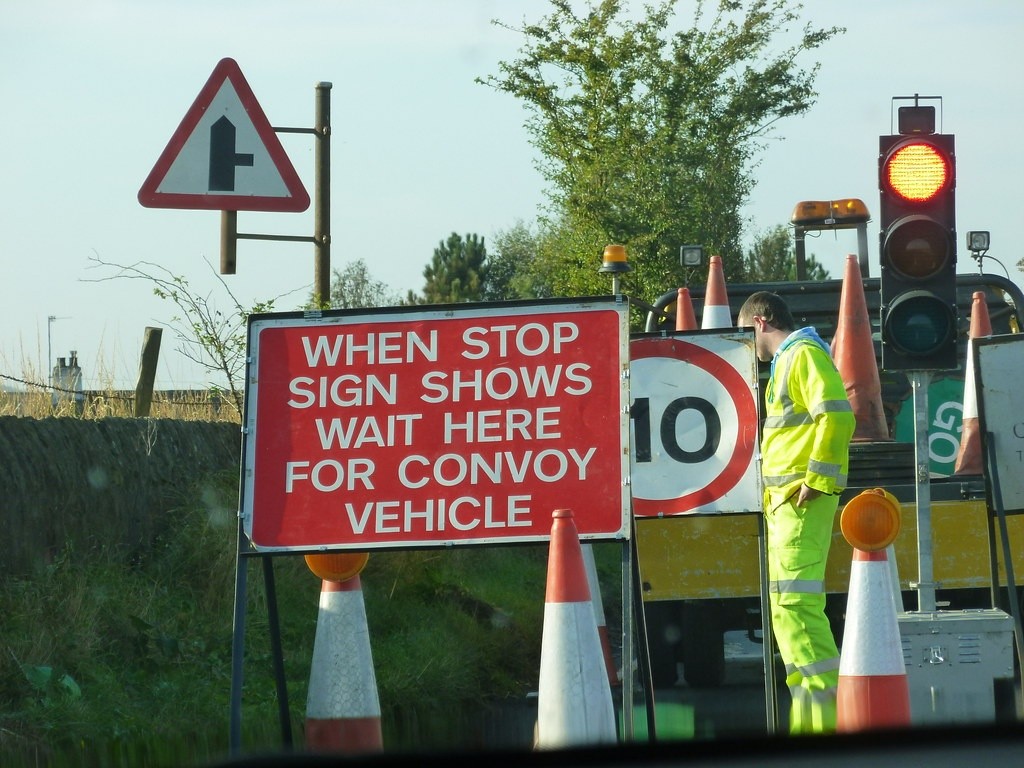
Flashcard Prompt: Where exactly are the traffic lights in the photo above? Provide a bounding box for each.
[880,134,965,372]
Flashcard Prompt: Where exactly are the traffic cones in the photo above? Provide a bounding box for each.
[697,253,734,335]
[835,542,915,733]
[829,254,893,445]
[954,290,998,482]
[524,539,618,703]
[304,569,386,758]
[534,510,620,751]
[678,289,699,332]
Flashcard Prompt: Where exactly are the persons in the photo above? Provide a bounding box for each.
[732,290,862,736]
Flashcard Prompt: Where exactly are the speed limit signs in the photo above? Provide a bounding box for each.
[628,331,766,516]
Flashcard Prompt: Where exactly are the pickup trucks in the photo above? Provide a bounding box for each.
[594,196,1024,682]
